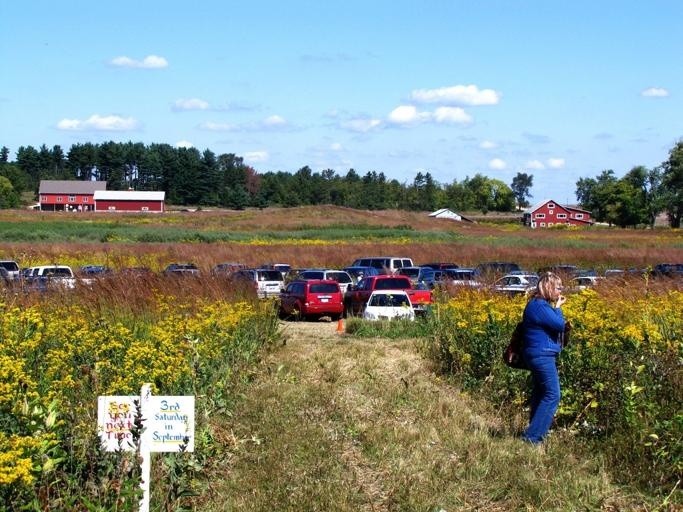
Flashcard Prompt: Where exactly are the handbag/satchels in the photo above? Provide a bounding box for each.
[504,321,573,372]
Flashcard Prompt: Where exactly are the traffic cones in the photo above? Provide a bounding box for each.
[336,320,344,332]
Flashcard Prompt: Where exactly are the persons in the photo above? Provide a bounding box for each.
[520,271,567,445]
